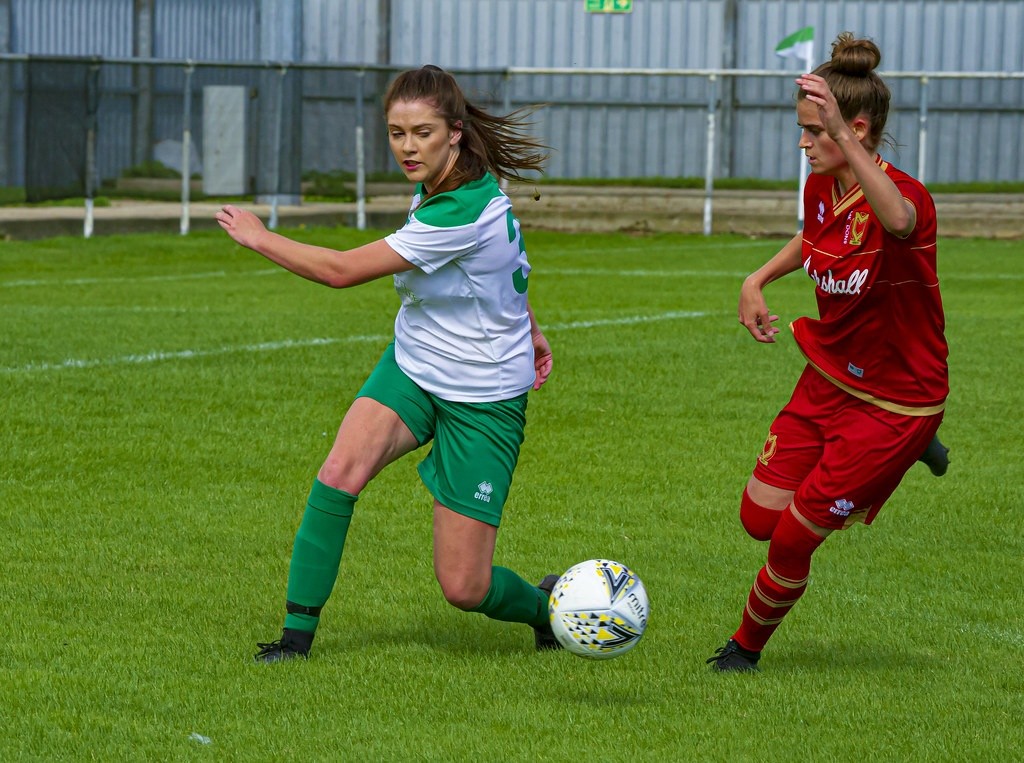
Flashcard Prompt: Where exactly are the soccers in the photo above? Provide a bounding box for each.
[546,558,649,659]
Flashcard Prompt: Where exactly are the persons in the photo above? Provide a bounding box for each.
[708,34,951,672]
[215,65,559,656]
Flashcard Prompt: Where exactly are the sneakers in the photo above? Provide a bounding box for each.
[535,574,560,651]
[706,639,762,674]
[254,639,303,660]
[919,434,951,477]
